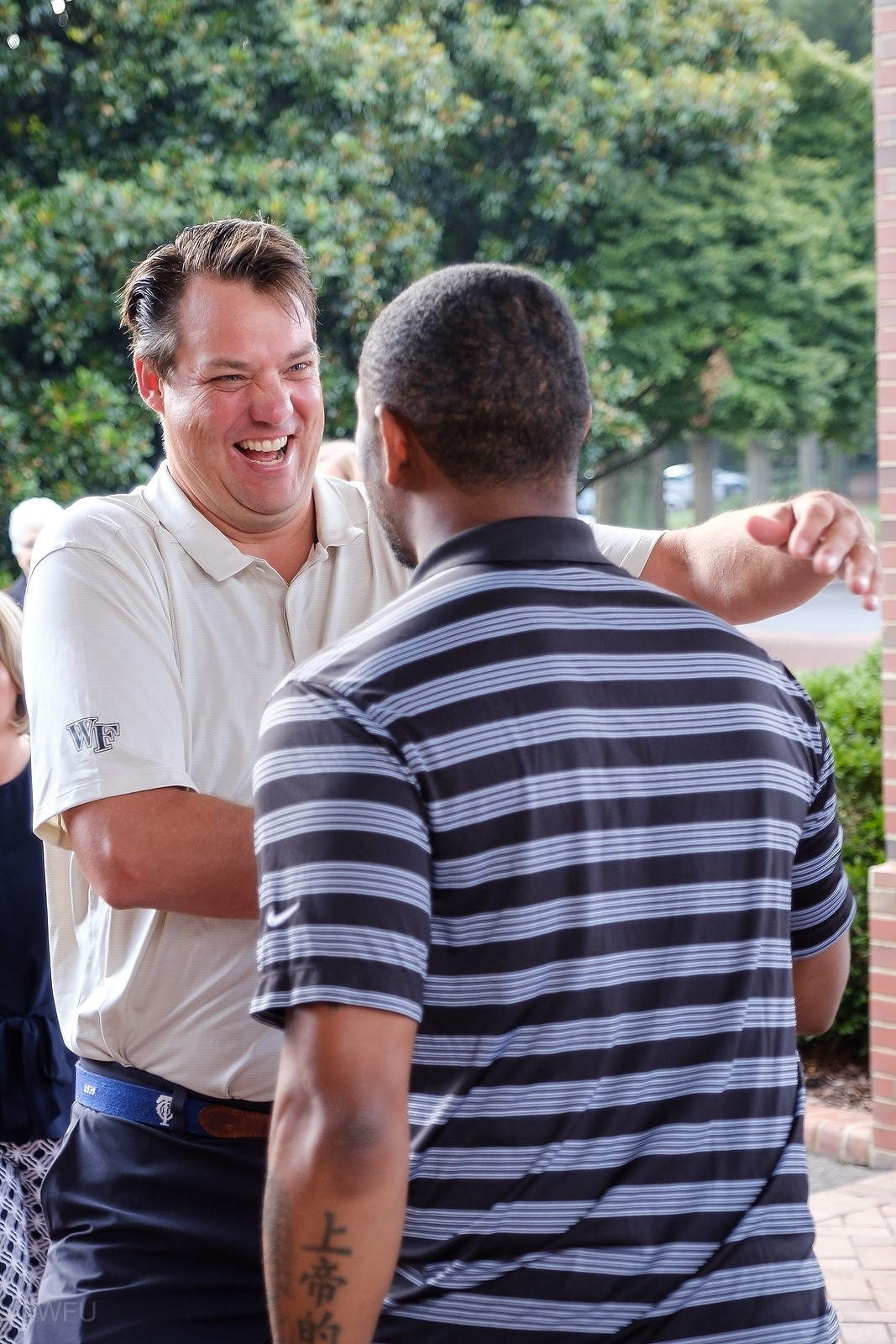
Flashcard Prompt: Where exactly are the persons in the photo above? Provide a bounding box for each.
[247,261,860,1343]
[0,496,77,1344]
[21,218,882,1344]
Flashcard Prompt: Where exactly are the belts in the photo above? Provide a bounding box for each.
[67,1060,273,1145]
